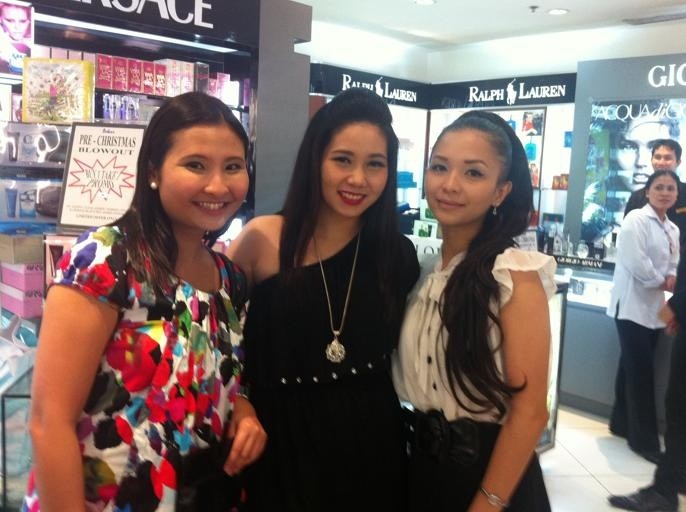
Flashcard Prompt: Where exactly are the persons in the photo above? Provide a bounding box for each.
[207,85,424,511]
[605,225,685,510]
[0,4,32,75]
[16,86,272,509]
[623,139,686,243]
[578,99,681,242]
[604,168,682,462]
[380,108,566,512]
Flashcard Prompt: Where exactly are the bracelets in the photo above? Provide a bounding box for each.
[475,483,510,509]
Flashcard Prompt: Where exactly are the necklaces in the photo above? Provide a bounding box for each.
[306,224,363,365]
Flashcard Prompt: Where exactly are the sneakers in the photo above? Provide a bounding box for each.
[609,424,665,466]
[609,486,679,512]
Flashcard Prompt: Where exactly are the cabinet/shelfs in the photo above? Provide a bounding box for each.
[1,6,258,389]
